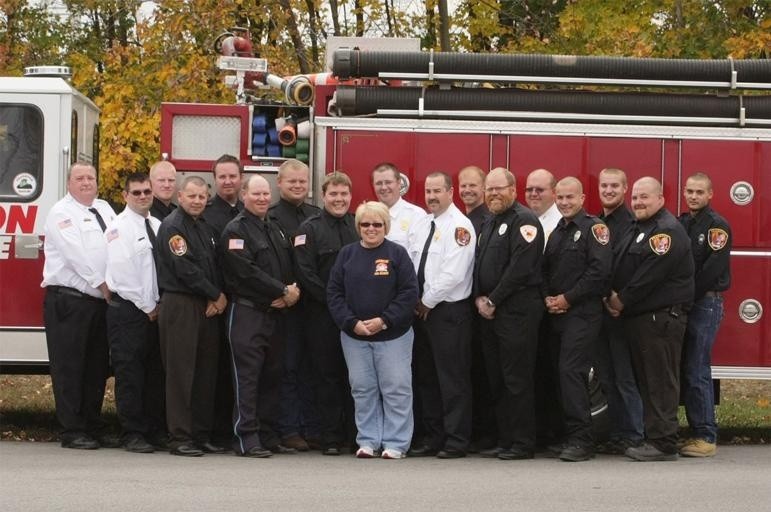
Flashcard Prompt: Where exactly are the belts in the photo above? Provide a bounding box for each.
[109,300,121,307]
[236,297,295,314]
[704,291,719,297]
[47,285,82,299]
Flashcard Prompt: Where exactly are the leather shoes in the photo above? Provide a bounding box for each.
[407,444,534,460]
[61,431,224,458]
[242,436,358,458]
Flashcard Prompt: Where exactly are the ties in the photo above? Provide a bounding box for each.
[417,220,435,298]
[87,207,105,232]
[144,218,163,304]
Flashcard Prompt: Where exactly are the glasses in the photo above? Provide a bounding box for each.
[524,187,551,192]
[359,223,384,227]
[127,189,151,195]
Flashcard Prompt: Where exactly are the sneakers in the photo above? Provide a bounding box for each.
[675,439,698,453]
[381,449,406,459]
[356,448,380,457]
[680,441,715,457]
[546,439,679,462]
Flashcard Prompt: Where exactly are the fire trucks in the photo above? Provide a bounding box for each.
[2,22,771,435]
